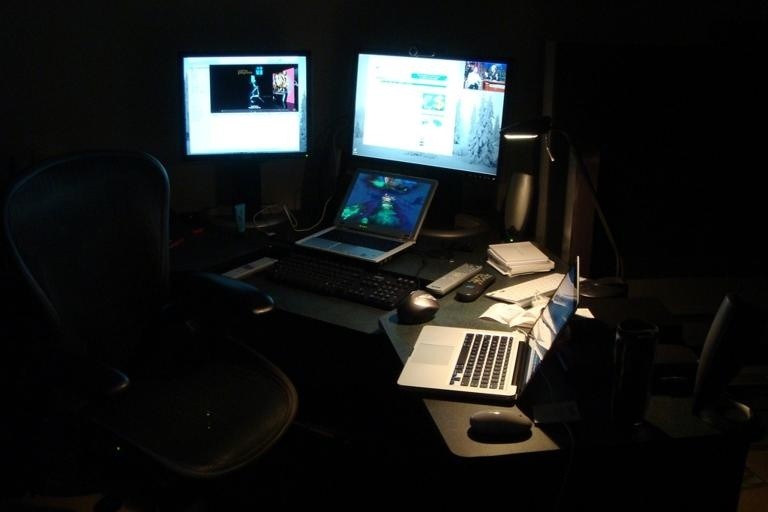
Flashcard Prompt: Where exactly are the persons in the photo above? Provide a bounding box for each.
[272,71,287,107]
[483,65,503,82]
[465,65,487,91]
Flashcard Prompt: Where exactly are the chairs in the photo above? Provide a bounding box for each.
[0,149,298,512]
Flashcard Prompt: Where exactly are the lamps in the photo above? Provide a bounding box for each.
[502,123,631,302]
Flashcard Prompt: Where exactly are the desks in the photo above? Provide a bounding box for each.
[377,272,757,508]
[182,217,500,410]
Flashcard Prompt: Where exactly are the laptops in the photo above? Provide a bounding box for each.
[398,255,581,402]
[295,164,440,270]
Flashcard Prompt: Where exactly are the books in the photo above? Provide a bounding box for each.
[484,271,588,308]
[486,241,557,279]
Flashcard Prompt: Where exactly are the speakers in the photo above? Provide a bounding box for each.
[504,173,537,240]
[687,288,755,433]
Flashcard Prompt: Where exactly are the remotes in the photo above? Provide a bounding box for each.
[425,264,483,297]
[457,272,496,302]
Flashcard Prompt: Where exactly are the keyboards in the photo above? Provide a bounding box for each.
[271,247,419,311]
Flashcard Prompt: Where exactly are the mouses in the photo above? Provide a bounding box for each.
[397,289,439,325]
[471,412,528,436]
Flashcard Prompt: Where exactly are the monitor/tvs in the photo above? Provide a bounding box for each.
[346,47,516,239]
[176,48,314,231]
